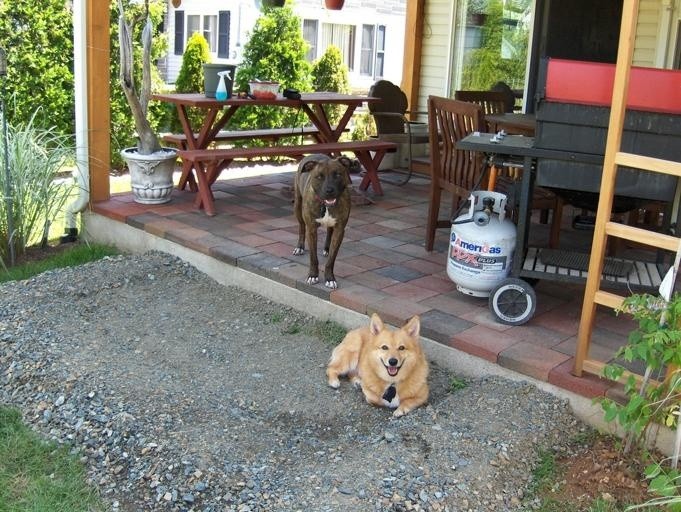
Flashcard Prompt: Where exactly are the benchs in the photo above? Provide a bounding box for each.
[161,125,397,219]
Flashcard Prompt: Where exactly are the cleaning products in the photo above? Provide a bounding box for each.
[216,70,233,100]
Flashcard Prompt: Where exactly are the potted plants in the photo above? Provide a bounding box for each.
[469,1,490,27]
[118,0,180,206]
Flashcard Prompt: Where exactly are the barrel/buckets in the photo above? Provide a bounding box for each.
[202,63,237,97]
[400,121,427,167]
[368,135,394,171]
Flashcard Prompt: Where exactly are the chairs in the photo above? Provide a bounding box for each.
[360,81,564,253]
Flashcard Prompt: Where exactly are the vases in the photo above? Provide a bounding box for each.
[326,0,345,10]
[248,81,281,100]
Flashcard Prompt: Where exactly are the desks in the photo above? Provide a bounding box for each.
[151,91,384,217]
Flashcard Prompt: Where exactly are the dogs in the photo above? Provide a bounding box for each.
[326,311,430,418]
[292,152,354,289]
